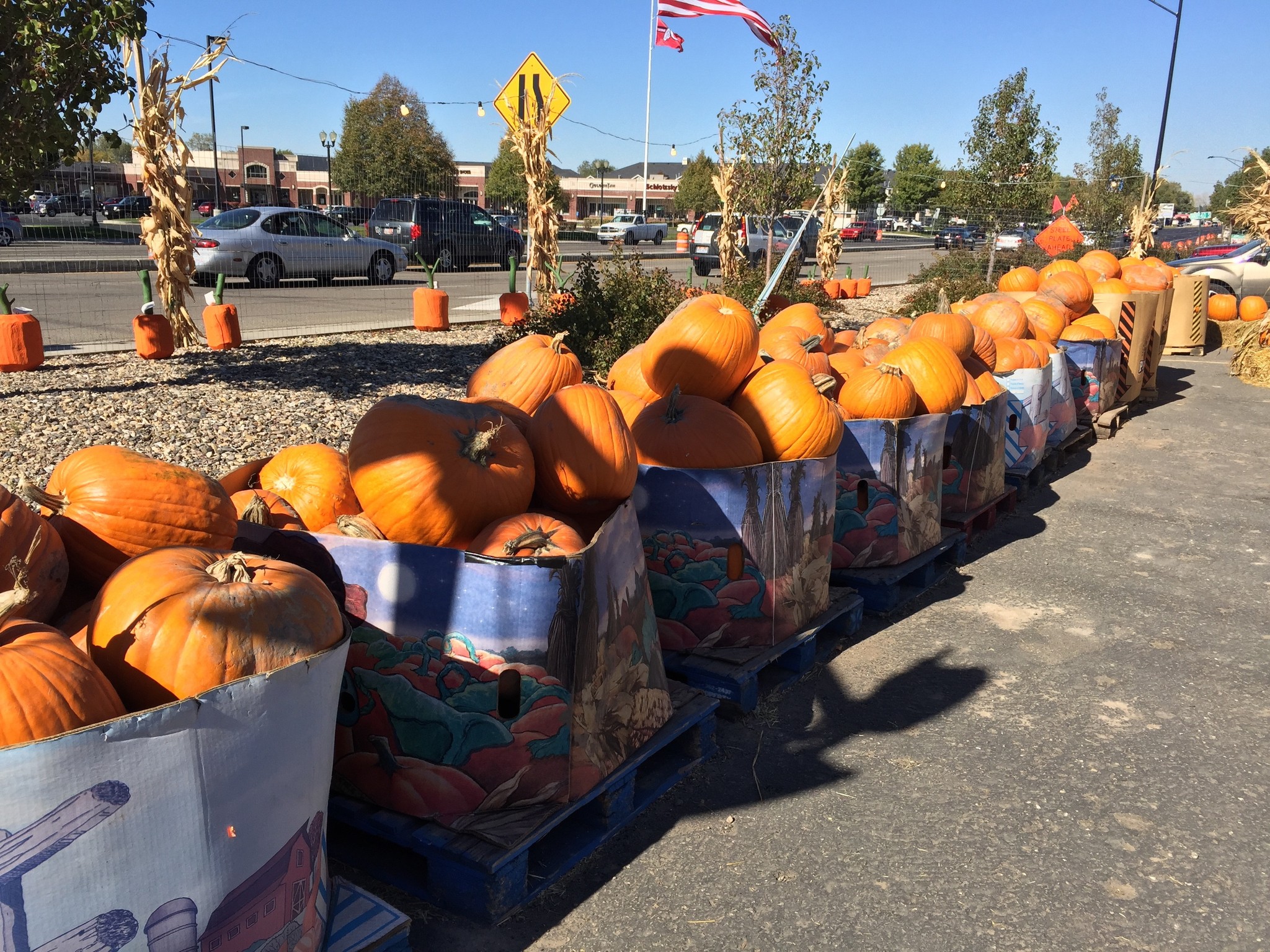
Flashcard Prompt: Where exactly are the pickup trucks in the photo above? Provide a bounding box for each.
[597,210,668,246]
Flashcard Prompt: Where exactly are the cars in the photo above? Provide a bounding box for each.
[191,198,376,226]
[839,221,877,242]
[676,220,699,234]
[1177,221,1192,227]
[1165,238,1270,310]
[1121,223,1160,243]
[995,228,1039,252]
[0,210,23,247]
[0,189,152,221]
[183,206,408,289]
[1079,230,1099,246]
[935,227,976,251]
[555,213,563,222]
[471,207,520,229]
[873,215,925,232]
[1211,222,1218,227]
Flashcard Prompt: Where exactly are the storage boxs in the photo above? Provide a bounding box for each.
[0,274,1211,952]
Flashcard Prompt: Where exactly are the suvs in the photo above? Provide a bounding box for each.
[777,213,839,266]
[365,198,525,273]
[690,211,801,277]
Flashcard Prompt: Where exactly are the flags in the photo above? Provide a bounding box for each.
[241,126,249,130]
[655,0,786,56]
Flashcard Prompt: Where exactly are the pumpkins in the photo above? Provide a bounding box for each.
[605,250,1182,471]
[1239,296,1268,321]
[231,329,636,556]
[1258,331,1270,346]
[0,444,343,748]
[1208,294,1237,321]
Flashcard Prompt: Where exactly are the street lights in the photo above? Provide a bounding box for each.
[595,160,610,227]
[241,125,249,205]
[319,131,337,233]
[1208,156,1245,187]
[80,106,100,227]
[206,34,226,210]
